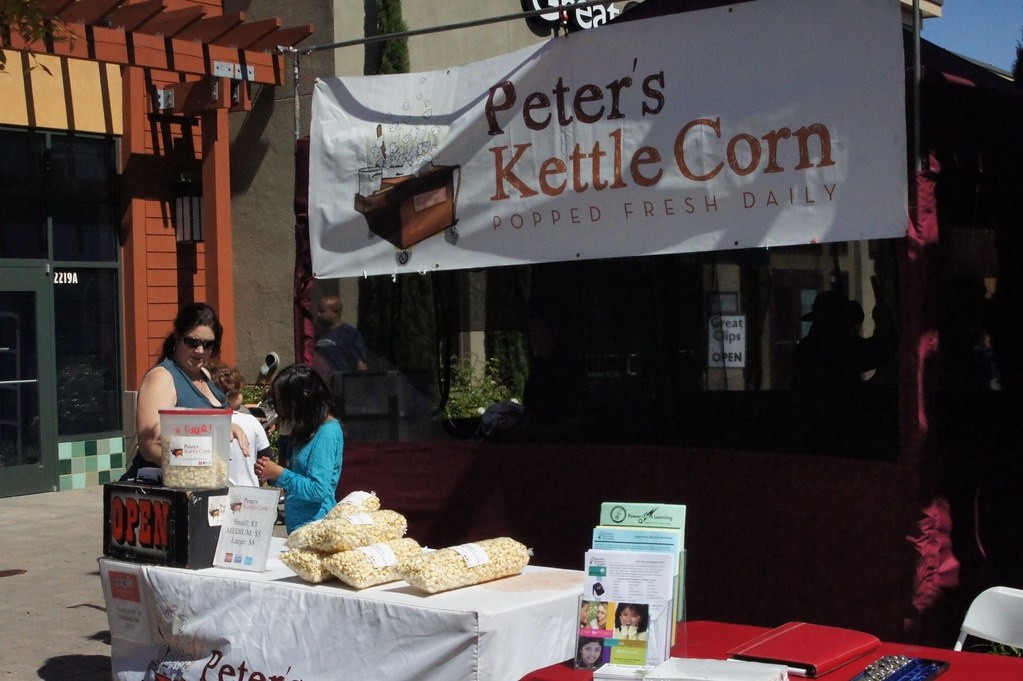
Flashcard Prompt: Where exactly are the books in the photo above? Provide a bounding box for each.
[726,622,881,677]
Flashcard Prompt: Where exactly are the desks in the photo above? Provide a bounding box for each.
[96,534,585,681]
[521,620,1023,681]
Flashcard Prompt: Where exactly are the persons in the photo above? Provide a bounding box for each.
[314,295,369,419]
[580,600,608,630]
[576,636,605,670]
[790,292,995,462]
[119,302,276,488]
[615,602,648,640]
[253,364,344,535]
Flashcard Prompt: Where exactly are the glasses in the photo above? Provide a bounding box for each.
[181,335,218,350]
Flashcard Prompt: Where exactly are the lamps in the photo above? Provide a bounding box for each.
[176,172,203,243]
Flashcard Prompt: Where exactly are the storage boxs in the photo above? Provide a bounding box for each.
[331,371,444,443]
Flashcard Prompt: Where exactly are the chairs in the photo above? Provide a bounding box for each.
[954,586,1023,653]
